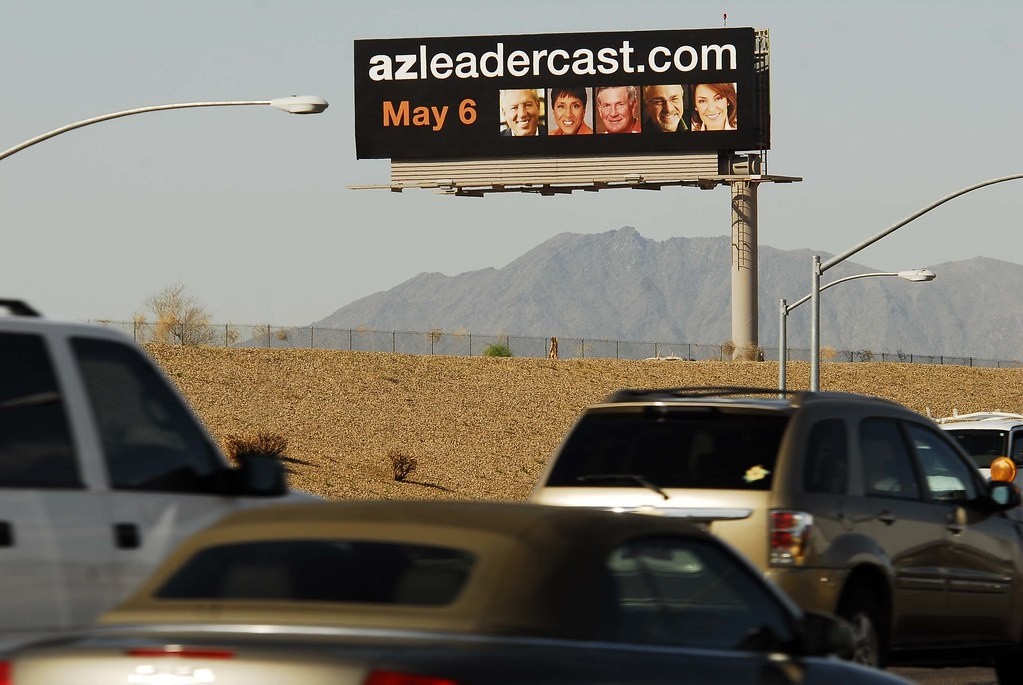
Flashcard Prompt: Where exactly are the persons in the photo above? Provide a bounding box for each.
[689,84,737,131]
[643,85,688,132]
[548,87,593,136]
[500,89,546,136]
[548,337,558,359]
[596,86,641,134]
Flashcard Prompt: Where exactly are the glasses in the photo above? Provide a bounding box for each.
[646,97,680,108]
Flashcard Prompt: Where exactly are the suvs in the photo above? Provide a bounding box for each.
[0,300,326,658]
[528,382,1023,683]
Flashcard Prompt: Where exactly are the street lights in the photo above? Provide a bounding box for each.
[777,268,938,396]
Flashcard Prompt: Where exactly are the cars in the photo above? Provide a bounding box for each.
[0,502,914,685]
[915,409,1023,495]
